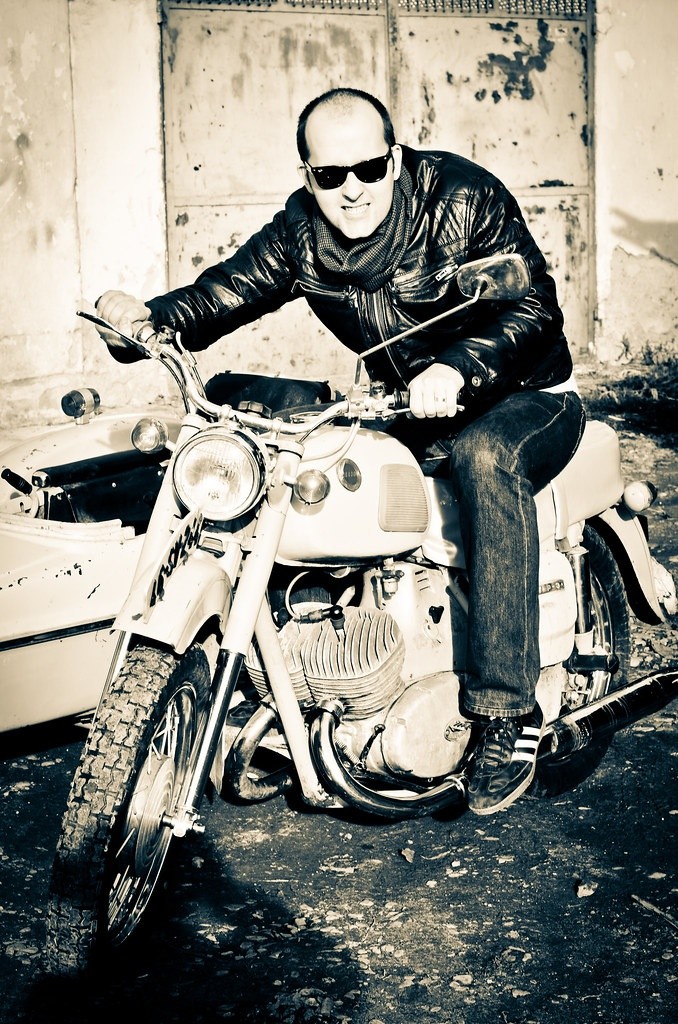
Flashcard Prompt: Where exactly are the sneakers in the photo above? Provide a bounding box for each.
[222,696,286,745]
[467,701,546,816]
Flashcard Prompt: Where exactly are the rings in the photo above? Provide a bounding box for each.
[435,397,446,401]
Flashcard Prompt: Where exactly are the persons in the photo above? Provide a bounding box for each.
[96,88,586,817]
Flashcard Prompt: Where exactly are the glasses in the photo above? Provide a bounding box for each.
[305,146,392,190]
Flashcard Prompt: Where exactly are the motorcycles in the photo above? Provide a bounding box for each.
[49,246,678,992]
